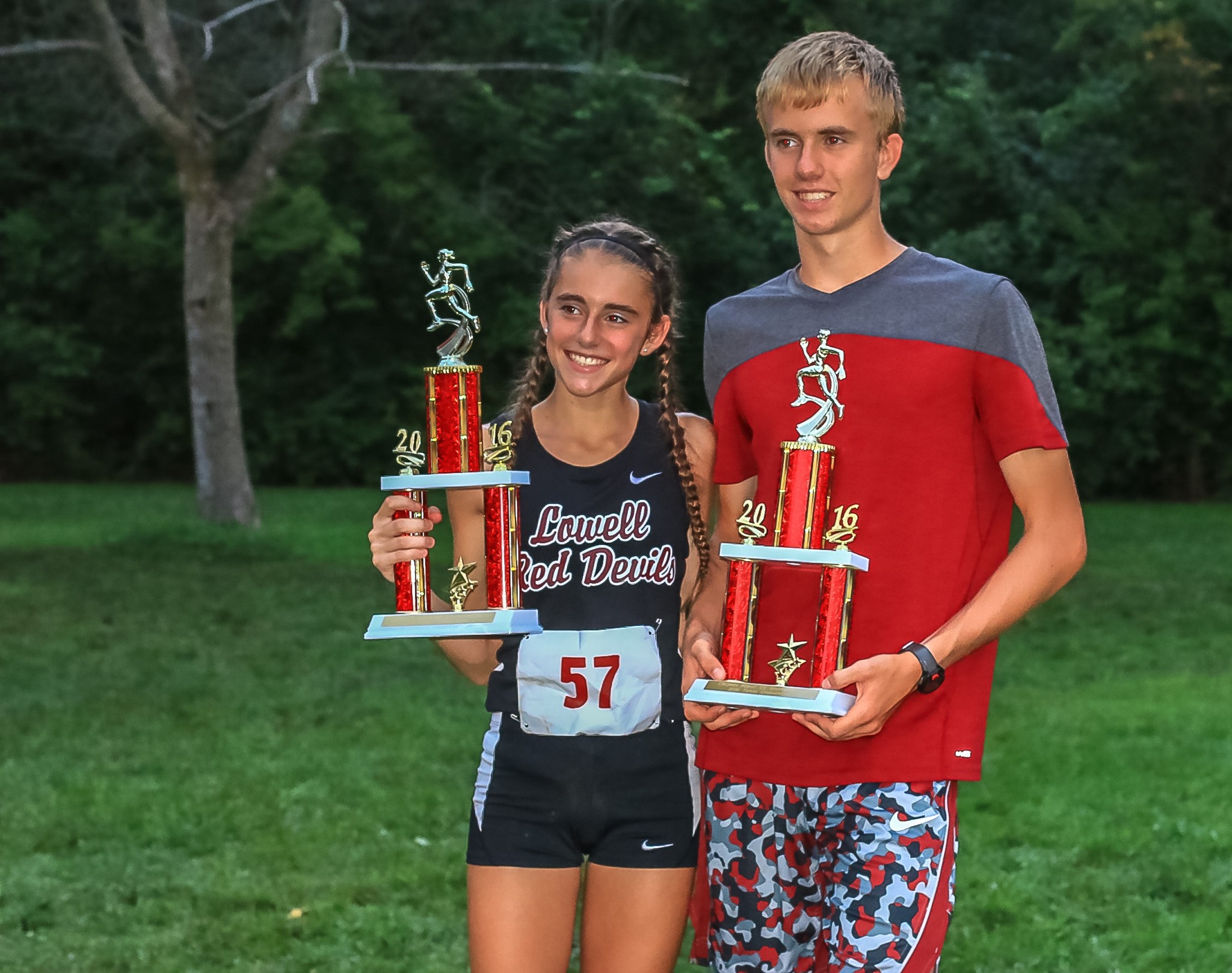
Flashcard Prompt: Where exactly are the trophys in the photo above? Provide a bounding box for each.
[363,248,544,642]
[687,330,870,719]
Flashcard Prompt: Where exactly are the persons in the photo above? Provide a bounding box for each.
[367,217,718,973]
[420,248,480,334]
[680,34,1085,973]
[791,328,847,418]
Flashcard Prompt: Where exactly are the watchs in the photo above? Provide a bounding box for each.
[898,641,946,695]
